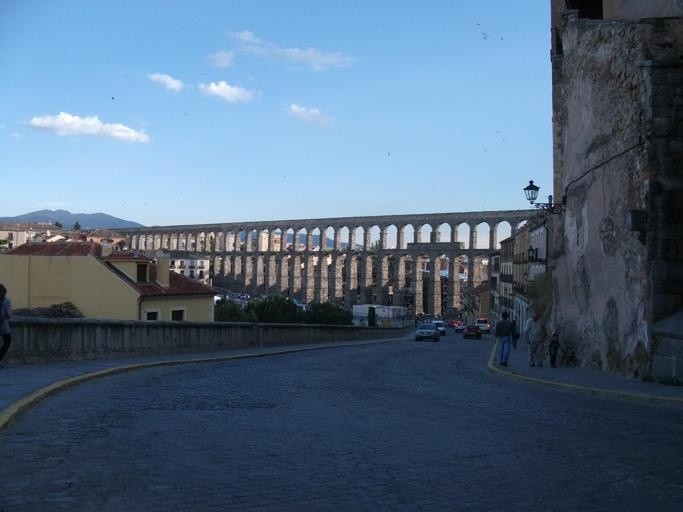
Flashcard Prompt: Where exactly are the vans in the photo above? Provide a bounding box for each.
[472,317,490,333]
[296,303,307,311]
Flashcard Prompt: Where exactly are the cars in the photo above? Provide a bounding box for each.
[239,294,249,300]
[452,320,460,329]
[413,323,440,342]
[461,324,481,340]
[448,320,453,328]
[453,322,466,332]
[431,320,447,337]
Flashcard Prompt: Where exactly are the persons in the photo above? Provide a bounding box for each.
[491,311,512,367]
[0,284,12,368]
[525,311,547,367]
[510,319,520,349]
[548,332,561,368]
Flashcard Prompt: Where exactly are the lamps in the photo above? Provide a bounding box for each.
[522,179,566,216]
